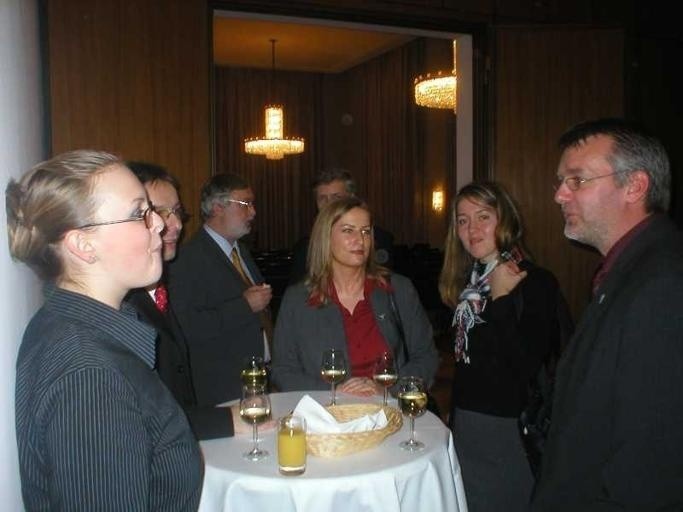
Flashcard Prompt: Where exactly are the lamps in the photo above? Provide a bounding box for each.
[243,38,305,161]
[414,70,457,114]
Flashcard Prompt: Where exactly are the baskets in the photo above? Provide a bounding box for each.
[276,403,403,457]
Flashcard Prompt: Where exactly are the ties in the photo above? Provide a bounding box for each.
[155,281,167,312]
[232,248,268,323]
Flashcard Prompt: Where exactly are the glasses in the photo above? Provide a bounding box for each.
[552,168,634,193]
[155,205,183,220]
[225,197,255,208]
[65,207,153,229]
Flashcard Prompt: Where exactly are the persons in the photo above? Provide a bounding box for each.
[166,173,272,411]
[270,194,437,400]
[126,160,279,440]
[530,115,683,512]
[439,181,559,512]
[4,149,205,512]
[287,170,415,283]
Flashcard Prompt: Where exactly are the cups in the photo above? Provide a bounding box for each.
[319,348,349,408]
[239,386,272,463]
[239,354,268,393]
[397,376,428,451]
[276,417,306,477]
[371,350,399,405]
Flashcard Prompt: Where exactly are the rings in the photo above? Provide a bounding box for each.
[360,376,366,385]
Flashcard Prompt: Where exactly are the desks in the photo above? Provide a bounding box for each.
[198,390,467,512]
